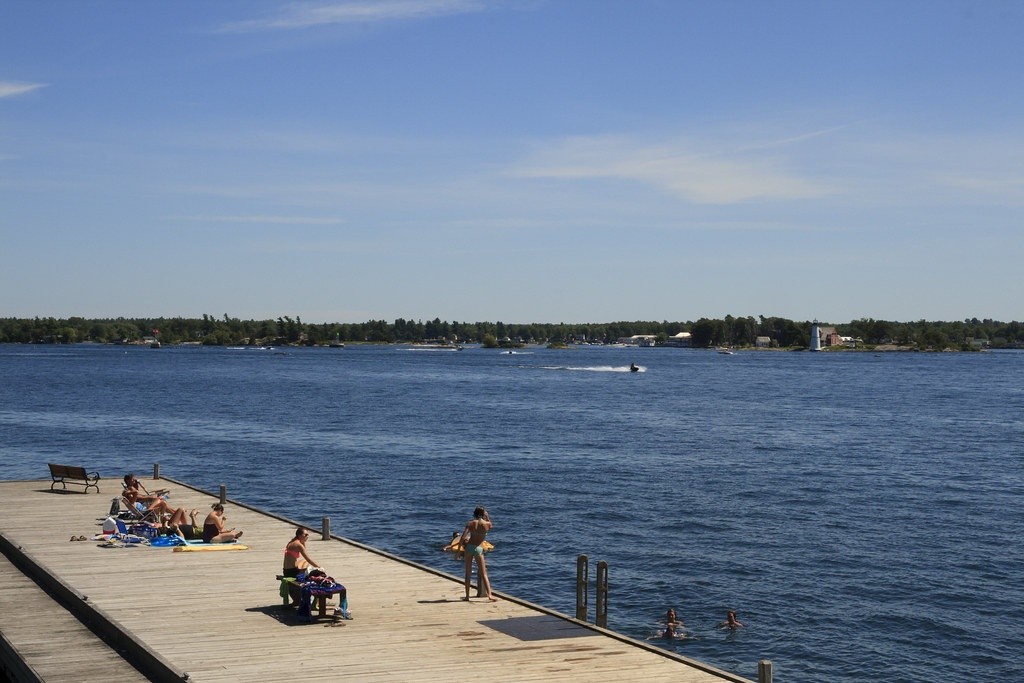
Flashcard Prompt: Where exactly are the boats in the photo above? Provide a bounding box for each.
[718,350,733,354]
[450,535,494,555]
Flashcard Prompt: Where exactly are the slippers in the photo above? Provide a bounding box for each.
[78,535,87,541]
[70,535,78,541]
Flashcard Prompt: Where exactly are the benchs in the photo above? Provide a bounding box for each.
[48,463,100,493]
[277,574,338,624]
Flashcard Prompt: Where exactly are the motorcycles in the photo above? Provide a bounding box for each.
[630,366,640,373]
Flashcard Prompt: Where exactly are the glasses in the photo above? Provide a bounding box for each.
[302,534,308,536]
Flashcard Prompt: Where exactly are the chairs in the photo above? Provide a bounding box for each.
[120,480,171,533]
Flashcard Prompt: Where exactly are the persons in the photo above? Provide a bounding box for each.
[124,475,157,498]
[454,506,498,602]
[204,504,243,543]
[666,623,676,636]
[122,489,174,517]
[154,508,235,544]
[667,609,676,621]
[283,527,319,610]
[443,532,460,550]
[725,610,742,626]
